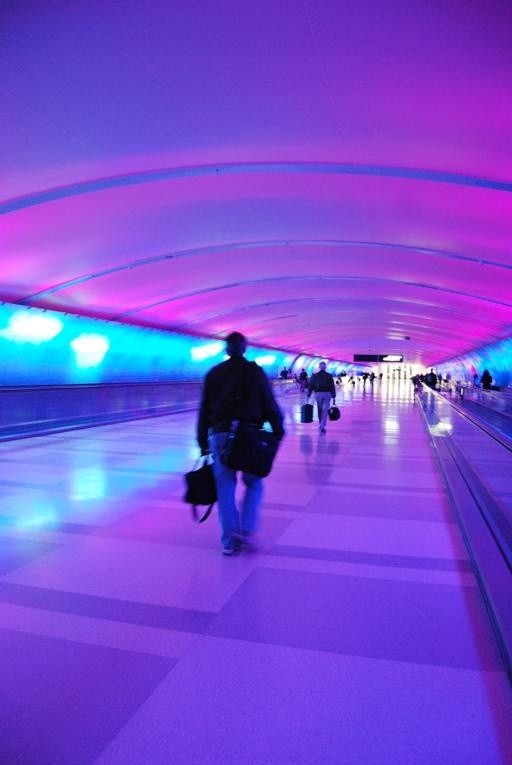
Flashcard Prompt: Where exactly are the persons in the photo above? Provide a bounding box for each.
[415,365,443,392]
[472,373,481,402]
[304,434,335,495]
[306,362,337,433]
[479,368,493,400]
[281,365,383,393]
[196,329,287,559]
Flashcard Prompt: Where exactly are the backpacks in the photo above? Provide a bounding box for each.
[315,373,331,392]
[427,374,435,384]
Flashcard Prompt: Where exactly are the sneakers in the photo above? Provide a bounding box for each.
[239,533,265,553]
[318,427,326,434]
[221,538,242,557]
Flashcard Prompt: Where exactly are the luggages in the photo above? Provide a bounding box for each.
[301,402,315,423]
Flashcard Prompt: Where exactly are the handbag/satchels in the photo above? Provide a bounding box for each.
[327,407,340,421]
[181,456,224,524]
[219,423,279,477]
